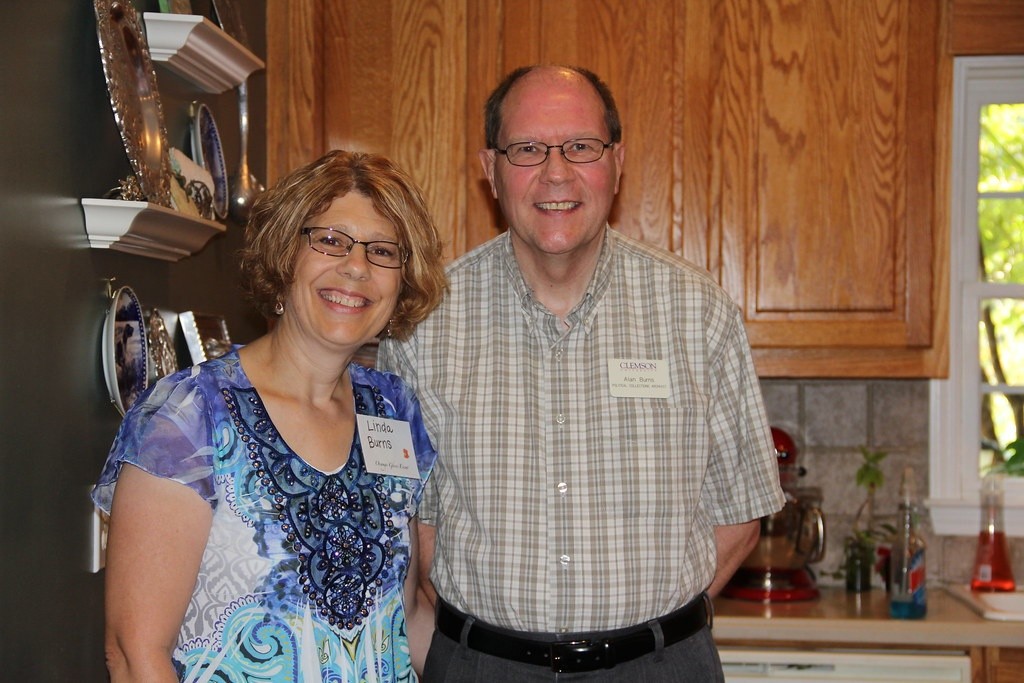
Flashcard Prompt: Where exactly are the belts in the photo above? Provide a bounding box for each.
[434,595,708,673]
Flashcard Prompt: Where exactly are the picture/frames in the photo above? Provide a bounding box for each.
[92,0,171,207]
[179,311,233,366]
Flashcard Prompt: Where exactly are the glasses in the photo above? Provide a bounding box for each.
[300,227,412,269]
[496,138,613,167]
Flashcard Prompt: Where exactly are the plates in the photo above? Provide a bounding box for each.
[191,103,230,221]
[101,284,150,419]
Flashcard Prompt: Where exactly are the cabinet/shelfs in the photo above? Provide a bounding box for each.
[265,0,950,376]
[81,11,266,261]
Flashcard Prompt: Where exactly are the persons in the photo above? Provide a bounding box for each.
[371,63,786,683]
[89,150,453,683]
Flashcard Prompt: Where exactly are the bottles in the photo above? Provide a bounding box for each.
[972,479,1017,592]
[888,468,927,618]
